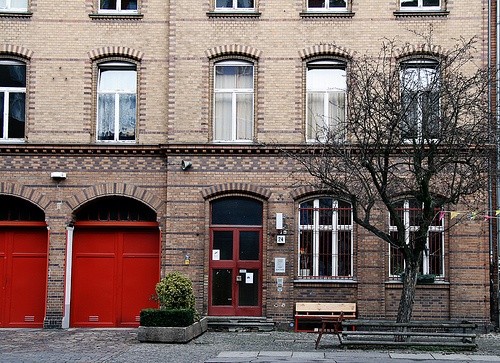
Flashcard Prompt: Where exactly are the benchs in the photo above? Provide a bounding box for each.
[315,312,343,348]
[294,302,356,332]
